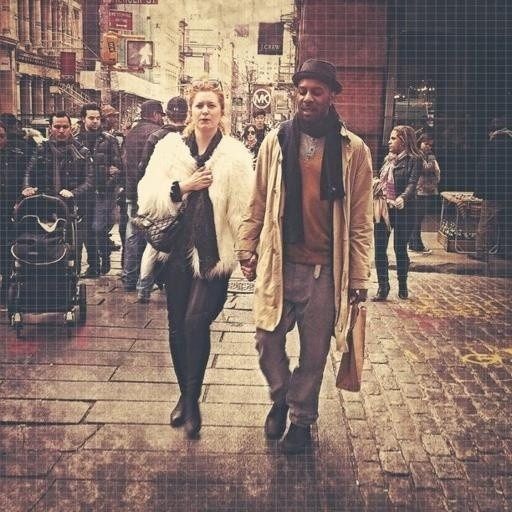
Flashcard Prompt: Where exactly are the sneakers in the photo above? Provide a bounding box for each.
[408,247,433,254]
[370,282,390,302]
[101,256,110,274]
[278,422,311,454]
[121,278,151,300]
[80,266,100,278]
[264,399,289,439]
[398,279,408,298]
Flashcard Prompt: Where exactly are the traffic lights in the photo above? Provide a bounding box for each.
[126,40,154,68]
[101,35,116,66]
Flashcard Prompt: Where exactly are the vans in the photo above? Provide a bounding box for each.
[28,117,79,139]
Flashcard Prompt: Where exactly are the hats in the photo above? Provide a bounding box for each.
[166,97,188,120]
[101,105,118,119]
[140,100,166,116]
[292,57,342,95]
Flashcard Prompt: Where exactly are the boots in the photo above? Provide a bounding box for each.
[169,312,211,437]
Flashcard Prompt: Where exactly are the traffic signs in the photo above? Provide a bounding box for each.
[107,8,134,33]
[95,0,158,5]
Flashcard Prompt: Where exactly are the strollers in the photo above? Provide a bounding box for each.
[4,187,90,338]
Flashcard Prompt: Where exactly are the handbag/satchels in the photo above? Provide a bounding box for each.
[142,215,183,253]
[336,302,367,392]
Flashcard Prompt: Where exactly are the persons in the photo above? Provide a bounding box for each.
[128,78,259,437]
[0,95,277,305]
[235,55,374,455]
[136,44,152,67]
[367,124,425,302]
[467,113,512,263]
[405,126,440,254]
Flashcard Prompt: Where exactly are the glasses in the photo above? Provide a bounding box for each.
[191,79,223,91]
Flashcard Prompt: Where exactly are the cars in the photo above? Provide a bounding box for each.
[20,125,45,145]
